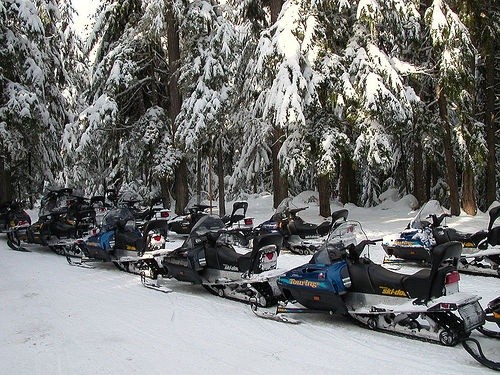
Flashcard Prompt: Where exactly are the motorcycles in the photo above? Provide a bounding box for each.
[381,200,500,278]
[462,294,500,372]
[141,214,283,308]
[251,221,486,346]
[258,198,348,255]
[63,207,168,280]
[90,190,157,223]
[8,189,101,259]
[167,191,255,248]
